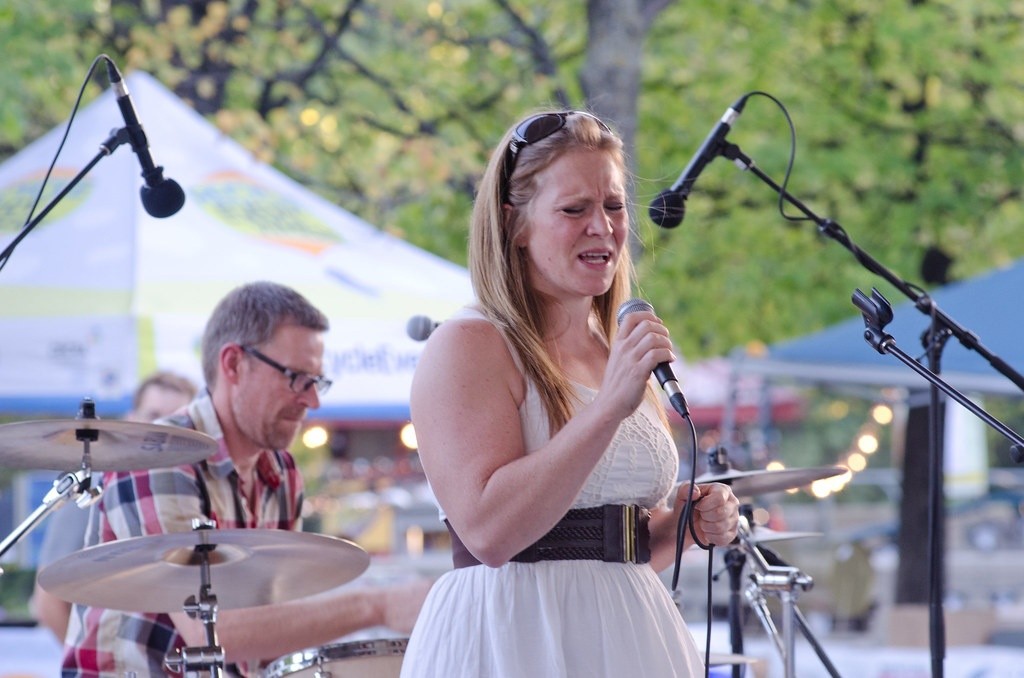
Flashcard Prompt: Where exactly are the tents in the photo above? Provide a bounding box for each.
[0,71,473,421]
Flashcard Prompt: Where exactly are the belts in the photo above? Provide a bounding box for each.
[444,505,652,565]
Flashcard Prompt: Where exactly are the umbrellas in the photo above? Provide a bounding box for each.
[749,259,1024,400]
[665,355,798,425]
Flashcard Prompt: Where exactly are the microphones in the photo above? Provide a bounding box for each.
[408,316,440,341]
[649,97,746,229]
[617,299,690,418]
[106,59,186,217]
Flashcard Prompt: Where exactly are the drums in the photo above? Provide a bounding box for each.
[263,636,407,678]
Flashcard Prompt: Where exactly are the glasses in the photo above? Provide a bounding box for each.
[500,111,612,200]
[243,342,331,395]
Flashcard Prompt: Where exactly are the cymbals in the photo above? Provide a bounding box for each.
[0,414,220,474]
[35,525,372,614]
[722,522,826,548]
[666,463,850,496]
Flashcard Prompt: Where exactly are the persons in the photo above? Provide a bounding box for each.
[62,281,429,678]
[29,373,193,646]
[400,111,741,678]
